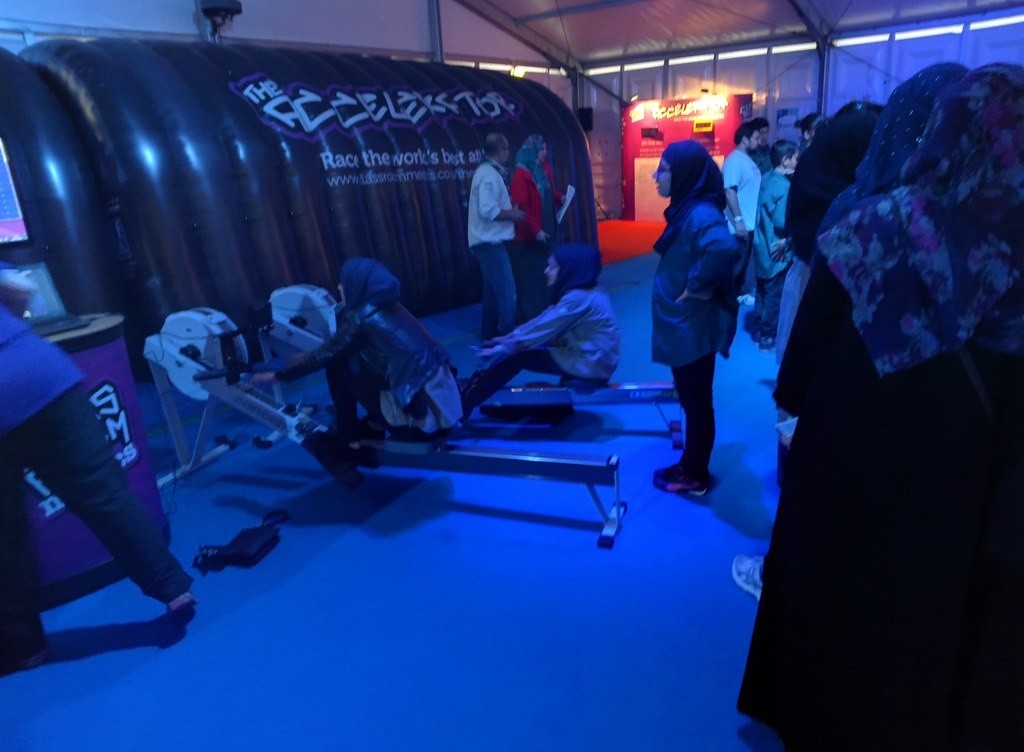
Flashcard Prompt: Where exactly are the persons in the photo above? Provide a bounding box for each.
[0,304,197,676]
[652,140,740,497]
[719,61,1024,574]
[468,132,526,340]
[510,134,566,280]
[248,255,464,470]
[458,244,620,426]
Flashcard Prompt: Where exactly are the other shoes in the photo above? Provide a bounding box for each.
[0,645,50,675]
[755,331,775,348]
[165,591,197,620]
[653,463,710,494]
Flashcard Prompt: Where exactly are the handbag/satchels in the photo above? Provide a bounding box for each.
[199,509,288,567]
